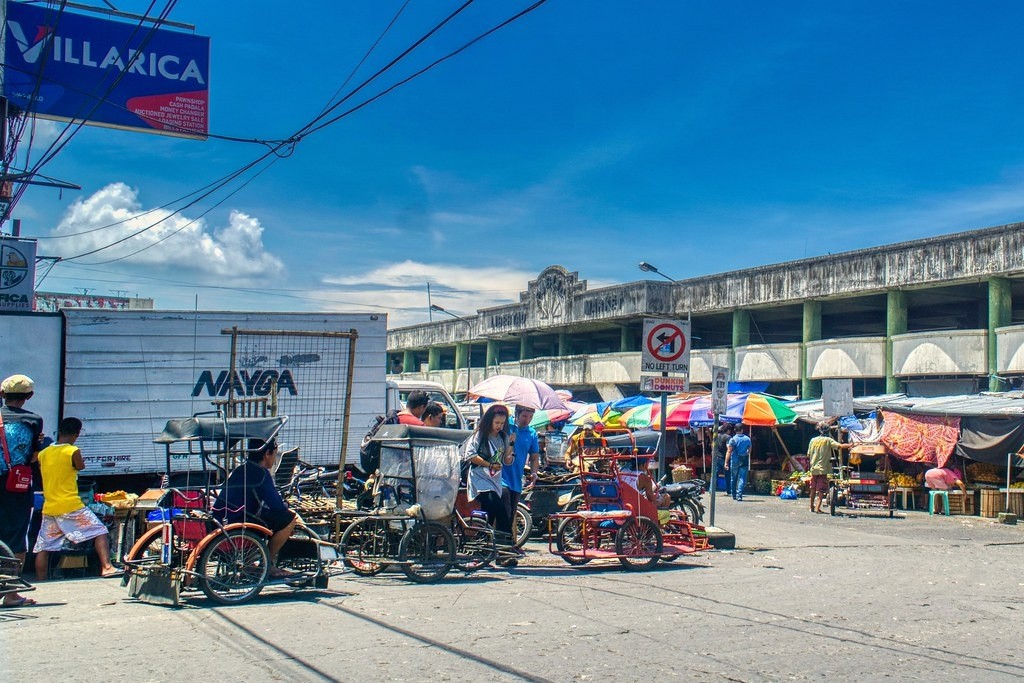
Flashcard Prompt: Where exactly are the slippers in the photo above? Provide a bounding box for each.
[103,568,125,577]
[0,597,37,608]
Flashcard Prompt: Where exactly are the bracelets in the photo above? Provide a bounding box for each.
[489,463,493,469]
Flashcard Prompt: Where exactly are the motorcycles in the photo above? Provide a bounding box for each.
[512,502,532,548]
[660,478,706,528]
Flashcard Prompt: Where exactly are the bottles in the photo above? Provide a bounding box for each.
[345,471,353,487]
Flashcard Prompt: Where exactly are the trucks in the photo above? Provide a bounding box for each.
[0,307,469,483]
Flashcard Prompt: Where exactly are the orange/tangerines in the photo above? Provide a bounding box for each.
[888,474,918,487]
[1009,481,1024,489]
[791,470,812,477]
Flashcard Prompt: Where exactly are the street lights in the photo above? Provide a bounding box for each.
[431,304,471,398]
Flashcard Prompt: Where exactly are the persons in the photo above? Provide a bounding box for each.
[565,417,609,474]
[398,391,443,427]
[717,427,732,495]
[807,425,862,513]
[725,424,752,500]
[0,374,43,605]
[614,451,671,530]
[33,417,123,580]
[212,438,299,577]
[925,466,968,514]
[464,403,539,566]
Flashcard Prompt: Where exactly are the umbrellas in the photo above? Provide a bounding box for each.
[468,375,568,446]
[513,390,799,475]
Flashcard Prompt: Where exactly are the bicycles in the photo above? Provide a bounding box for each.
[279,459,364,498]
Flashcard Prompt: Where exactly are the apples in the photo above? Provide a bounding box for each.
[966,461,1001,482]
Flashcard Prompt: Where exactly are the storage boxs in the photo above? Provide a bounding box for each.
[55,553,88,568]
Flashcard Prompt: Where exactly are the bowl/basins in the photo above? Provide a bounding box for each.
[76,479,96,491]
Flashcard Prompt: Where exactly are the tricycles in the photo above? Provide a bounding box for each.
[548,427,715,570]
[340,420,523,583]
[124,406,357,605]
[826,439,897,518]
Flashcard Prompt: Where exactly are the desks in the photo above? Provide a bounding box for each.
[889,487,916,511]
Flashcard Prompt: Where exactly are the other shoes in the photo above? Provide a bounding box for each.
[497,556,519,567]
[733,496,742,501]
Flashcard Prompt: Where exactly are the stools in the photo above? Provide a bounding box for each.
[929,490,950,516]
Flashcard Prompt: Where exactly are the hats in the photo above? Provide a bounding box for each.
[582,417,596,430]
[0,374,34,394]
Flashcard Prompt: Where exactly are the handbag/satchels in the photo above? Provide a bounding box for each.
[738,455,750,467]
[5,464,32,493]
[470,433,492,469]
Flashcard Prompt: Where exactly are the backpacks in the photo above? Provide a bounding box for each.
[359,409,402,473]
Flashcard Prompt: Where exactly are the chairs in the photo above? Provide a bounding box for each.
[166,488,267,552]
[846,471,888,494]
[50,501,118,580]
[584,479,659,532]
